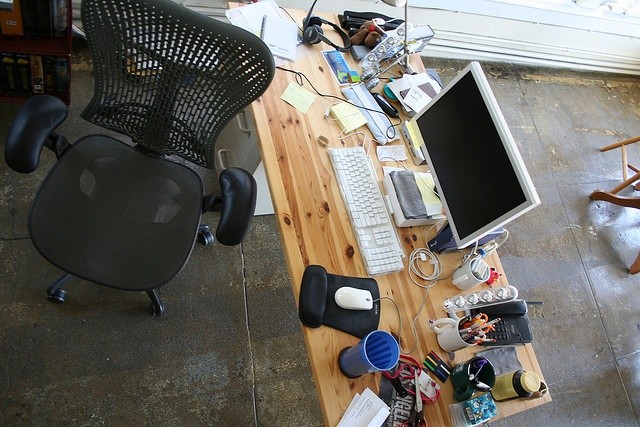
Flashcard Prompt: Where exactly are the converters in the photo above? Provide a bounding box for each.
[372,92,399,119]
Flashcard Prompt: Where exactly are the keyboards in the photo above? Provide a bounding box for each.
[326,146,405,276]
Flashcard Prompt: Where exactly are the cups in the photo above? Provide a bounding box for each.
[451,257,491,290]
[450,355,495,401]
[431,314,480,352]
[339,329,401,377]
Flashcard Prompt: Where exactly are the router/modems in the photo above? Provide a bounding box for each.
[399,120,427,166]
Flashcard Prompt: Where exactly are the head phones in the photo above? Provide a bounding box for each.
[302,13,351,52]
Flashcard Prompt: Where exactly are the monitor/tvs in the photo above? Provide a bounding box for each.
[383,60,543,251]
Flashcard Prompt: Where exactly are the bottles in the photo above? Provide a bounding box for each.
[490,369,541,402]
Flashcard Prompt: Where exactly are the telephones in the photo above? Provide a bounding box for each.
[463,299,533,345]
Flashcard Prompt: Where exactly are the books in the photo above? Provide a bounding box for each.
[52,0,67,38]
[259,14,299,63]
[2,53,69,97]
[400,82,437,110]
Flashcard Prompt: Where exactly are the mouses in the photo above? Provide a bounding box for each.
[334,287,374,311]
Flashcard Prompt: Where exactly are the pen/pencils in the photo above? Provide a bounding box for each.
[468,359,492,390]
[259,14,267,40]
[460,313,501,343]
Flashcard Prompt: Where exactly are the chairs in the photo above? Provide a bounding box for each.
[4,0,277,319]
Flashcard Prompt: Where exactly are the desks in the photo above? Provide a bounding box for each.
[225,0,555,427]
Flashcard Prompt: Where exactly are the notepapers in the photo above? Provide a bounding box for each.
[331,99,369,134]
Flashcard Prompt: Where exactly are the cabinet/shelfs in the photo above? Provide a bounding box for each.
[213,103,276,216]
[0,0,74,107]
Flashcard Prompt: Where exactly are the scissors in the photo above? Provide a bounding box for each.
[402,369,427,427]
